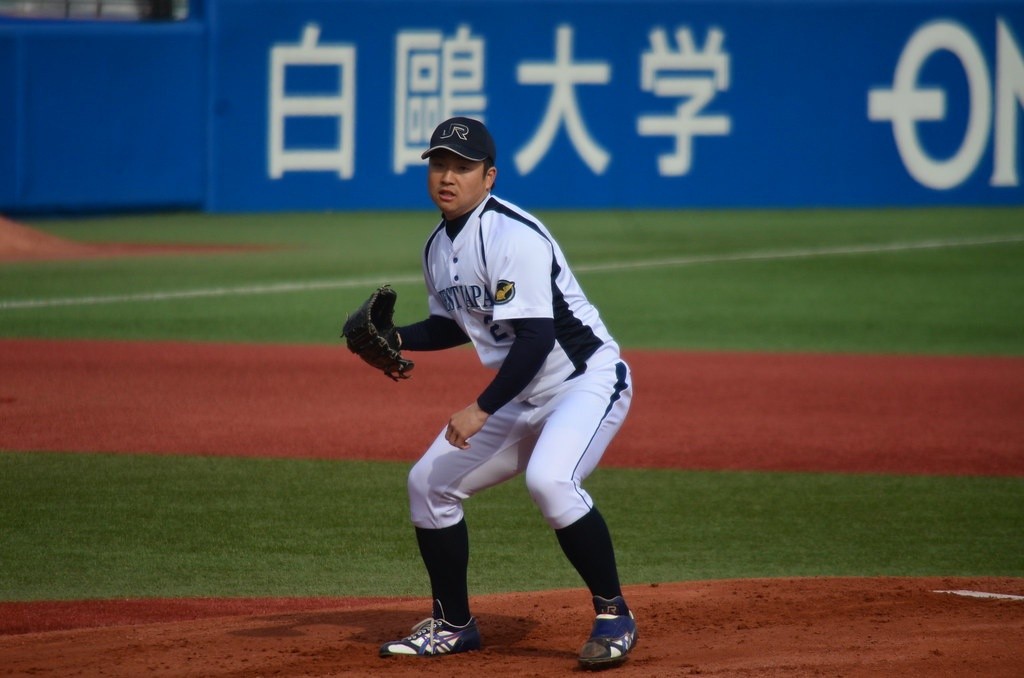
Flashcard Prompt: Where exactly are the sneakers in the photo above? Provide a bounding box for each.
[378,616,481,658]
[579,596,639,664]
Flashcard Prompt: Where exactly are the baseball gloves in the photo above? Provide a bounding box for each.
[340,285,414,383]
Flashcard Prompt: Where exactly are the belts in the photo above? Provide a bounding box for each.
[564,358,587,380]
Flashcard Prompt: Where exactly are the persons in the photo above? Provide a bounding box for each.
[378,120,637,661]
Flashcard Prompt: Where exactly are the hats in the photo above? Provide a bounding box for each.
[421,117,497,162]
